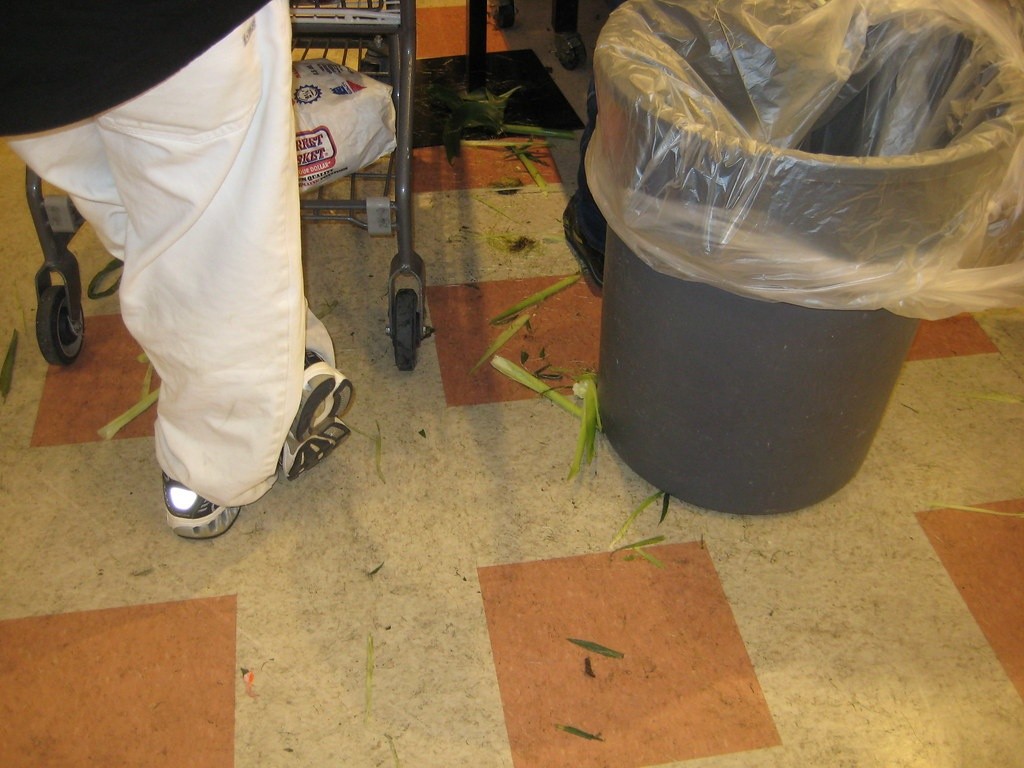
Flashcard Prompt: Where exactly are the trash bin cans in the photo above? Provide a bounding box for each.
[584,0,1024,516]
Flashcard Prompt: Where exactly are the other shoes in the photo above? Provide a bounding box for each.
[562,192,603,299]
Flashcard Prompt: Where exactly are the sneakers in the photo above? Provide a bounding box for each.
[161,468,242,540]
[279,349,353,483]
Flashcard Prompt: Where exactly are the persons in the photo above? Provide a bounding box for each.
[564,80,607,286]
[0,0,355,535]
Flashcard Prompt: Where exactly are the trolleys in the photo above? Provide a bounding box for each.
[25,0,435,373]
